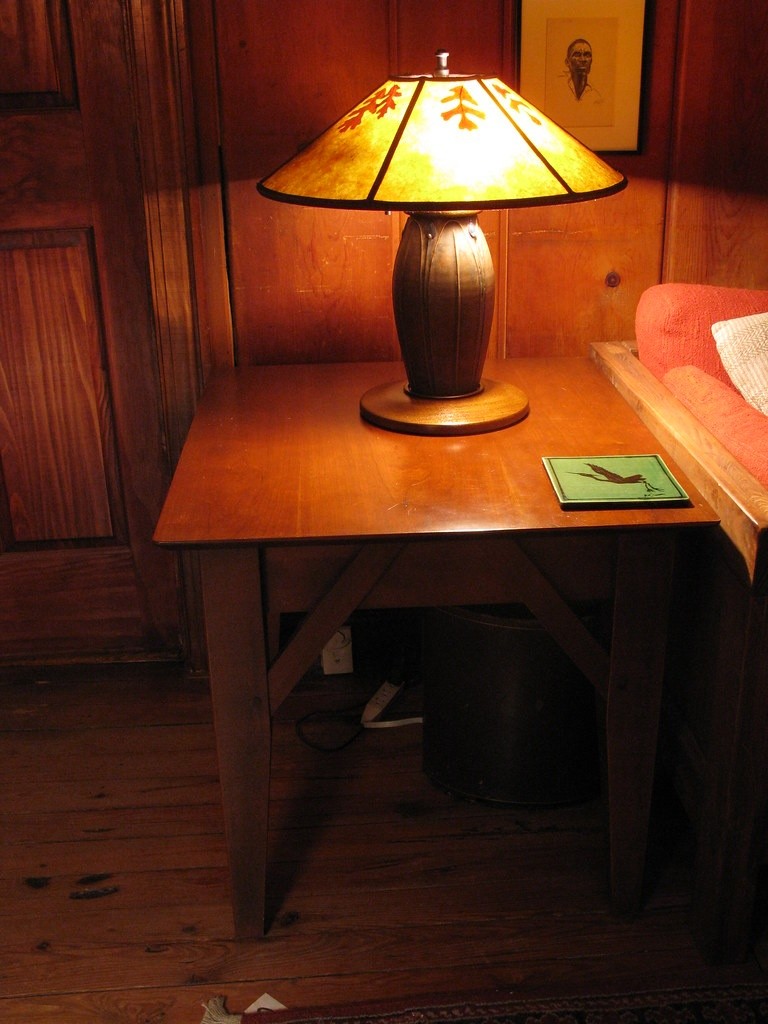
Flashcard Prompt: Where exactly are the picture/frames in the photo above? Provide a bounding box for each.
[513,0,658,159]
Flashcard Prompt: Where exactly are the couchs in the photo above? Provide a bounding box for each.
[584,342,768,969]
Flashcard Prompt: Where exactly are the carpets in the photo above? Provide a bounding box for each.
[242,975,768,1024]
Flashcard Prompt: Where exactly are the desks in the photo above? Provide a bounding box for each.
[152,354,723,943]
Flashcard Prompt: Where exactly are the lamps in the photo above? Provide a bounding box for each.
[254,51,629,436]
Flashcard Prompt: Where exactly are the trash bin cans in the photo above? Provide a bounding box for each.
[422,606,612,808]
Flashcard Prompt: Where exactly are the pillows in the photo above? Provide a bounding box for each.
[633,283,767,403]
[710,311,767,416]
